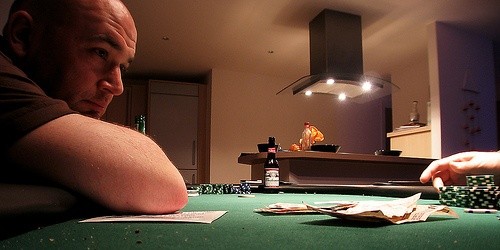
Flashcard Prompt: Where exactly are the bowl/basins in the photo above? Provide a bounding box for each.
[311,144,342,153]
[375,150,403,157]
[256,143,279,153]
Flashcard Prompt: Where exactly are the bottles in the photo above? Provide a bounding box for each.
[264,136,280,193]
[135,113,151,136]
[409,100,420,123]
[301,122,312,151]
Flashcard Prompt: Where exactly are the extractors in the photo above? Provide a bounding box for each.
[277,9,399,105]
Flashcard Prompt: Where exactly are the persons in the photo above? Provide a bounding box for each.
[302,122,311,150]
[419,150,500,193]
[0,0,188,214]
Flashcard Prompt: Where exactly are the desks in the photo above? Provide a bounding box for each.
[237,152,433,184]
[0,185,500,250]
